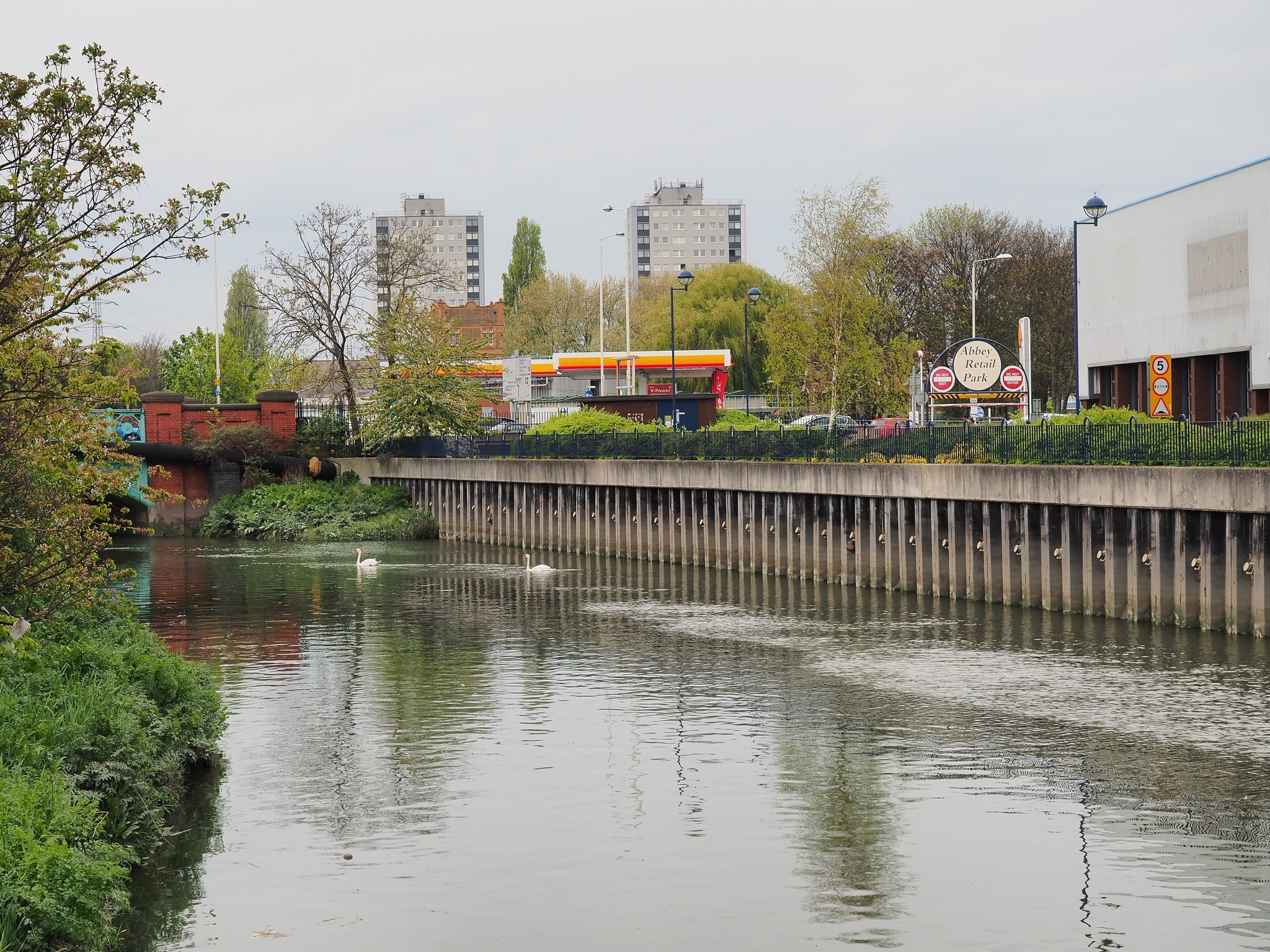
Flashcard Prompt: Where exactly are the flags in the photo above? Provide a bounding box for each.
[711,368,728,409]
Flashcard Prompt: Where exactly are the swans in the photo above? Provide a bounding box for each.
[354,548,382,566]
[523,554,556,571]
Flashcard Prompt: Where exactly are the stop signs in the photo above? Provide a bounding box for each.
[1000,365,1026,392]
[929,366,956,392]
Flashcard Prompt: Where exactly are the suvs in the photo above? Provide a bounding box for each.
[783,414,859,430]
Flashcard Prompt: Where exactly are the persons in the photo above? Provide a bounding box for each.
[963,415,972,424]
[969,418,975,424]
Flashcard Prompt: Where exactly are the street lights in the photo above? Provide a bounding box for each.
[917,350,926,426]
[744,284,761,419]
[927,362,938,426]
[670,265,692,430]
[1074,192,1108,416]
[601,205,641,397]
[601,231,626,398]
[972,253,1013,341]
[213,211,230,405]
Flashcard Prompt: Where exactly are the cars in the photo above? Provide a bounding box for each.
[1003,413,1076,426]
[472,417,528,436]
[869,418,922,439]
